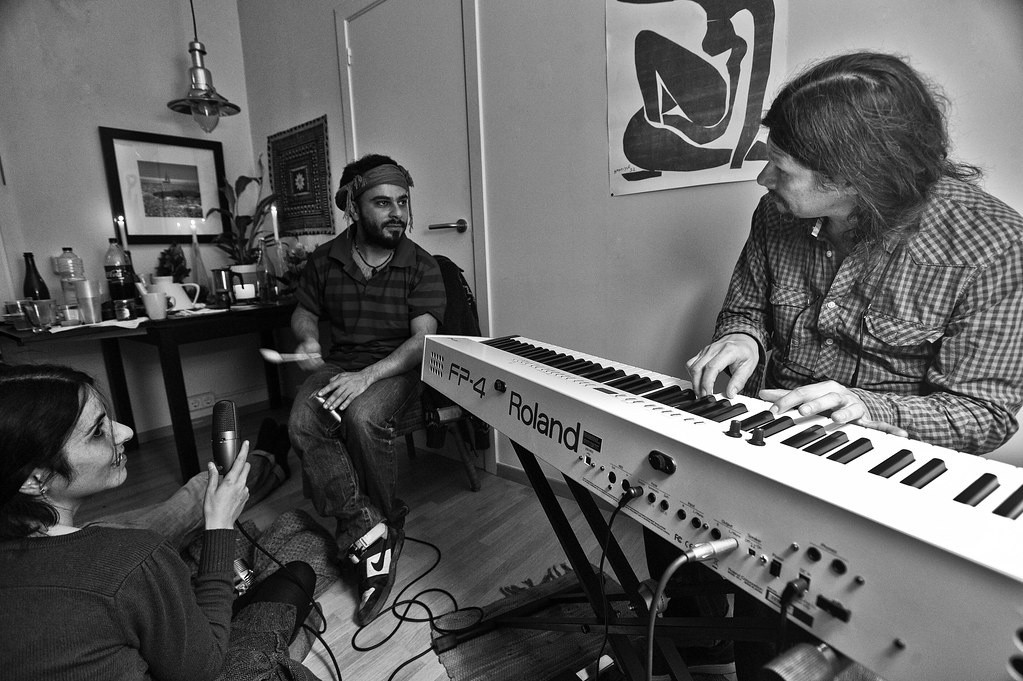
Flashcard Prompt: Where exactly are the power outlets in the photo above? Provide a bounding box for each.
[187,391,217,412]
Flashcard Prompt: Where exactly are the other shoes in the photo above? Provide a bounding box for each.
[612,637,736,681]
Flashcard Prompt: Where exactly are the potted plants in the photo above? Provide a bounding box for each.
[154,243,192,292]
[206,153,306,305]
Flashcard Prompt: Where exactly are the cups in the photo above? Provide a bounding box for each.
[24,300,56,334]
[4,296,34,331]
[59,304,79,320]
[140,292,176,320]
[74,279,102,324]
[304,386,343,438]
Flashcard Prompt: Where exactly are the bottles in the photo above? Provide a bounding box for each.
[57,247,86,305]
[214,289,231,308]
[22,251,52,299]
[256,241,277,305]
[104,237,140,317]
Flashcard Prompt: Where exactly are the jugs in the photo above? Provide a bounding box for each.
[134,275,201,310]
[210,267,245,304]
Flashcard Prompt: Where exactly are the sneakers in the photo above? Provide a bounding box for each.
[342,505,410,627]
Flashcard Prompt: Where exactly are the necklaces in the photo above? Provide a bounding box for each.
[353,228,396,277]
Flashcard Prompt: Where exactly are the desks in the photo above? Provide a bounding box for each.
[0,296,331,484]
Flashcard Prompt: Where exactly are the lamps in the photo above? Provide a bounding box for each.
[166,0,242,133]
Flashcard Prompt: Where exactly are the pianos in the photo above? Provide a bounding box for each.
[420,334,1023,681]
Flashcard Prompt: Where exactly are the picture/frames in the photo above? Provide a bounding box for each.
[98,125,234,246]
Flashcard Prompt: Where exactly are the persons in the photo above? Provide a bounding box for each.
[0,361,323,681]
[287,153,448,627]
[640,50,1023,681]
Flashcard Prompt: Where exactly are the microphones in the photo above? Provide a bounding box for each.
[212,399,239,484]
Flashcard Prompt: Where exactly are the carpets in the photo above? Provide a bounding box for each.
[429,561,726,681]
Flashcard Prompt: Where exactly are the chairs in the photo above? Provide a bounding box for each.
[301,256,490,501]
